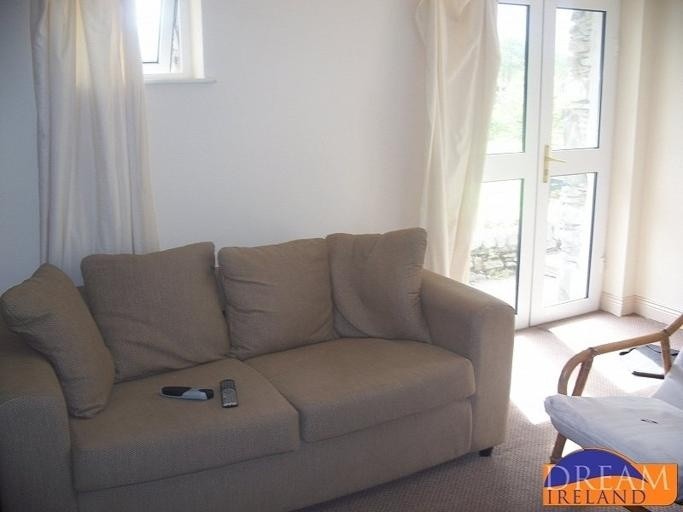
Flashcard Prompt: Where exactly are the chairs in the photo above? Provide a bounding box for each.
[543,309,683,512]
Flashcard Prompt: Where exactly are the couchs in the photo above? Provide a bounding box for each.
[1,265,515,510]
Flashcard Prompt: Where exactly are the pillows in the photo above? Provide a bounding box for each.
[2,228,428,422]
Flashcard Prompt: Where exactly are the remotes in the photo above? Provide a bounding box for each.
[159,384,214,400]
[220,379,238,408]
